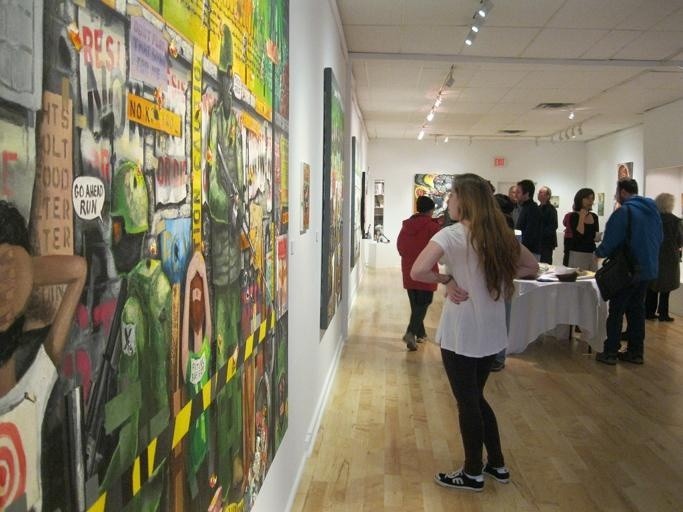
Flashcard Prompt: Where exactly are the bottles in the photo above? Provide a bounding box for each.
[552,247,564,267]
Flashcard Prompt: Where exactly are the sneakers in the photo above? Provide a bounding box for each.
[596,351,617,364]
[618,351,643,364]
[647,314,658,319]
[482,464,509,484]
[659,315,675,321]
[417,333,427,343]
[490,358,505,371]
[434,469,484,491]
[404,332,416,350]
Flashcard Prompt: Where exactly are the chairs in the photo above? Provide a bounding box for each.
[568,250,598,353]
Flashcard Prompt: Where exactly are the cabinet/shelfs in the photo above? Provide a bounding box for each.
[375,180,384,226]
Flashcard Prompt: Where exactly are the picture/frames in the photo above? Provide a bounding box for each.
[350,136,360,267]
[320,67,342,342]
[362,171,371,239]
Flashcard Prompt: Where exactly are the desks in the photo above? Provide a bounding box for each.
[503,263,607,355]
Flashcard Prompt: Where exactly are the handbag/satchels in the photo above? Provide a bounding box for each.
[595,253,640,301]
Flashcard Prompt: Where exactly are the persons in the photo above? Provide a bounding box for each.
[408,172,540,491]
[494,178,559,266]
[567,188,603,273]
[396,195,443,352]
[562,203,575,266]
[618,165,629,178]
[644,192,683,322]
[594,177,664,365]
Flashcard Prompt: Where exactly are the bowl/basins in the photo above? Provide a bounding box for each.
[557,274,577,281]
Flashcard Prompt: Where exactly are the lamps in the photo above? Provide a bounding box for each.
[478,1,494,20]
[418,68,454,140]
[559,124,583,140]
[465,30,477,47]
[471,19,482,33]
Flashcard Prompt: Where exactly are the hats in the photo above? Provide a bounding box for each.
[495,194,513,213]
[417,196,434,212]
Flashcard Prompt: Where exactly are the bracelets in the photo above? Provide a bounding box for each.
[442,275,453,285]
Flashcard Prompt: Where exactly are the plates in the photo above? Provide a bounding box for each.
[538,277,558,282]
[581,276,595,279]
